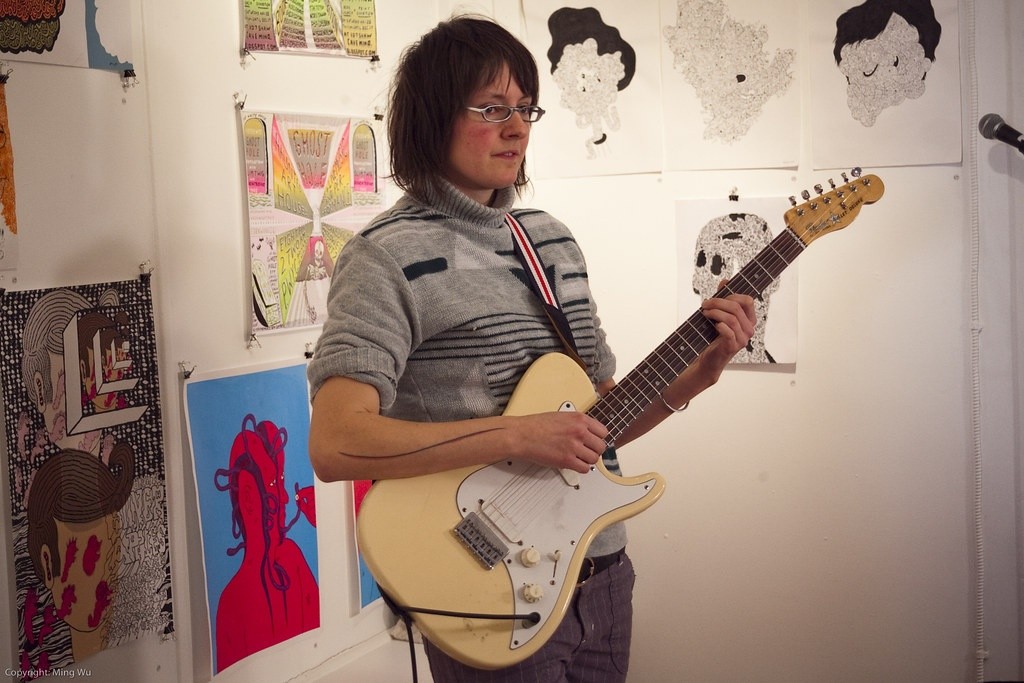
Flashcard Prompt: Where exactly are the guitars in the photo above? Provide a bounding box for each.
[353,170,889,671]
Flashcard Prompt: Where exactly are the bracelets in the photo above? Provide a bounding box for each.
[659,389,688,413]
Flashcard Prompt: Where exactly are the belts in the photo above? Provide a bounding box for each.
[576,546,625,588]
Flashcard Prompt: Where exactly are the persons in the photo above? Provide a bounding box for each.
[309,15,757,682]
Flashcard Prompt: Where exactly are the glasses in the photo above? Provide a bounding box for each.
[464,104,545,123]
